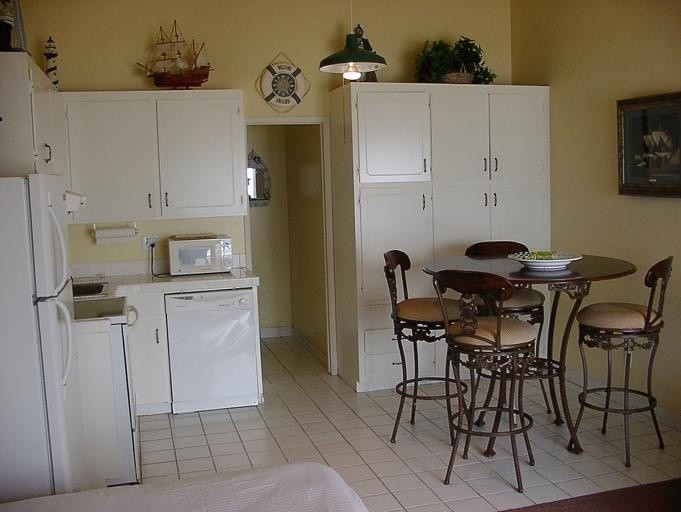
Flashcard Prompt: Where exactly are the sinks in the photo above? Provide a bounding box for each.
[72,281,107,299]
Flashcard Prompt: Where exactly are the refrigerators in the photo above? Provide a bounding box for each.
[0,173,81,501]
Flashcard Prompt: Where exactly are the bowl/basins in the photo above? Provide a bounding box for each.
[508,267,582,281]
[507,252,583,270]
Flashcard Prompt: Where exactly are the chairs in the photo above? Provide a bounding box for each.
[431,268,540,494]
[465,240,552,425]
[565,255,676,469]
[382,251,472,446]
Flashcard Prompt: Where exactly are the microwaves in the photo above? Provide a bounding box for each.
[166,234,233,277]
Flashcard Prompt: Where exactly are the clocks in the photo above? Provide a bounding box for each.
[256,52,312,114]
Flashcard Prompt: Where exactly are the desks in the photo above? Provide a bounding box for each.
[422,253,638,459]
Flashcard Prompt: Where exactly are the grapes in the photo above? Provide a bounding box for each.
[526,251,552,259]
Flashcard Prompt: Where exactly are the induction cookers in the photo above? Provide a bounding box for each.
[73,296,125,321]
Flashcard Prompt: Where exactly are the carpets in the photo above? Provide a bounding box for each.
[496,476,680,511]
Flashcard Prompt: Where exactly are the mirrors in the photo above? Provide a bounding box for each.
[247,149,274,208]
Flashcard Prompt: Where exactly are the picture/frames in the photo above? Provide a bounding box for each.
[614,90,681,199]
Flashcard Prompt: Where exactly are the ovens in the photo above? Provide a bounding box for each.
[114,303,144,485]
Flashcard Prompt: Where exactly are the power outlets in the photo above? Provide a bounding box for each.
[144,236,161,250]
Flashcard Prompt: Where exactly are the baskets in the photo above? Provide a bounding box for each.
[440,63,474,83]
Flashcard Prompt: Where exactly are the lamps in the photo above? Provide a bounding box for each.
[317,0,387,82]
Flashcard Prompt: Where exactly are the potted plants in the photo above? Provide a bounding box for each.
[416,35,499,85]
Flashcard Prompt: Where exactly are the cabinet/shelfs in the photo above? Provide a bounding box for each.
[122,294,173,420]
[352,80,434,185]
[435,185,550,382]
[356,185,435,300]
[358,301,436,394]
[0,49,66,184]
[432,81,555,181]
[64,89,246,219]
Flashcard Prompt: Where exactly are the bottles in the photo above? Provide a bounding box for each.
[44,36,60,90]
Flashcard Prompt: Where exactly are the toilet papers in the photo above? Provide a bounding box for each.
[94,227,135,245]
